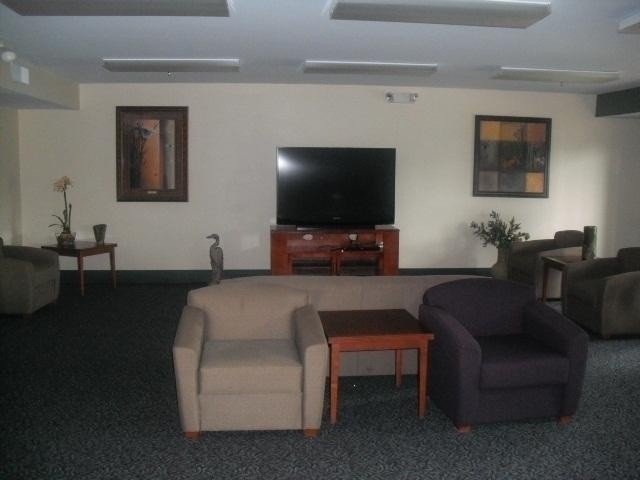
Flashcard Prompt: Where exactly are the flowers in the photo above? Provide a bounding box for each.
[48,175,73,233]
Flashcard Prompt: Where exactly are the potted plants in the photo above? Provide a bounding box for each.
[469,211,530,280]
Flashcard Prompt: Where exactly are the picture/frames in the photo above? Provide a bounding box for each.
[115,106,188,202]
[474,115,552,198]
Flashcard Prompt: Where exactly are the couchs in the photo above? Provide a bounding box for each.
[221,276,491,377]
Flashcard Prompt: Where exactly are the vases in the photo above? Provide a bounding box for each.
[57,232,74,244]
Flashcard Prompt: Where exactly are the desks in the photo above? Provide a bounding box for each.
[41,240,117,296]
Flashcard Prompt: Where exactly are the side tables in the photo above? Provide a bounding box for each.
[318,309,434,424]
[541,255,590,315]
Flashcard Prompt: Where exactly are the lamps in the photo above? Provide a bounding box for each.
[386,92,418,104]
[103,58,239,72]
[498,67,620,84]
[302,61,437,78]
[0,0,233,17]
[325,0,552,28]
[618,12,640,36]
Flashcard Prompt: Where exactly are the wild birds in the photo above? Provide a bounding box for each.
[206,234,223,284]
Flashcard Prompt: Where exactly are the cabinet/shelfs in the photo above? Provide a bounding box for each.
[269,225,399,276]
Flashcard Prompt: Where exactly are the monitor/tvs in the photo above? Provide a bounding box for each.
[274,146,397,230]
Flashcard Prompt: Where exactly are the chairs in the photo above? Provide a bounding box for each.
[419,279,588,432]
[0,237,60,317]
[172,283,327,436]
[561,247,640,339]
[508,230,584,300]
